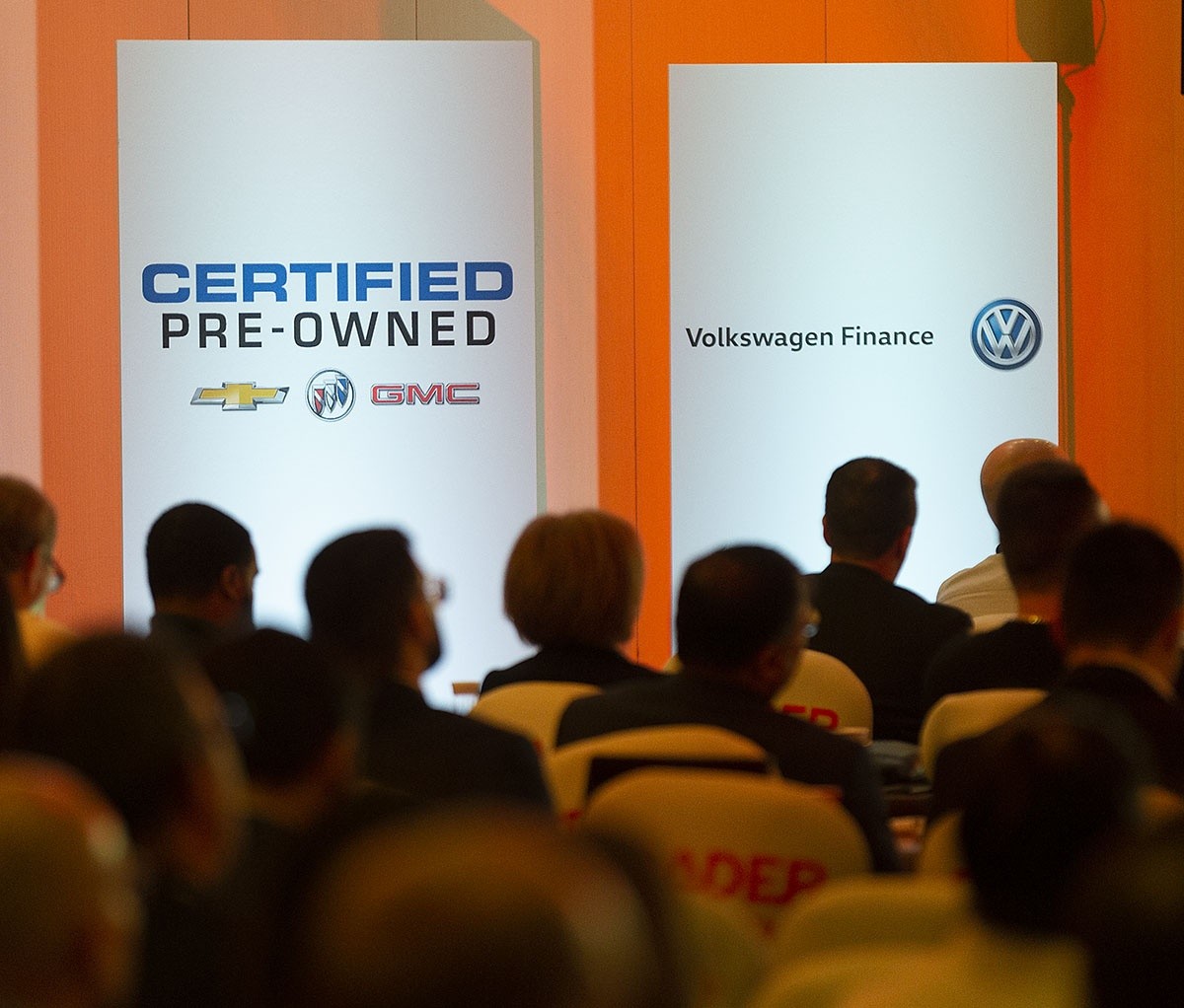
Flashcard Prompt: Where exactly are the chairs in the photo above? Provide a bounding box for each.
[0,614,1184,1008]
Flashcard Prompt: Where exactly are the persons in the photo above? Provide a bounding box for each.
[1,438,1183,1008]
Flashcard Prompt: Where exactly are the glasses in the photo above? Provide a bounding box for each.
[802,612,821,639]
[422,577,448,605]
[46,556,66,593]
